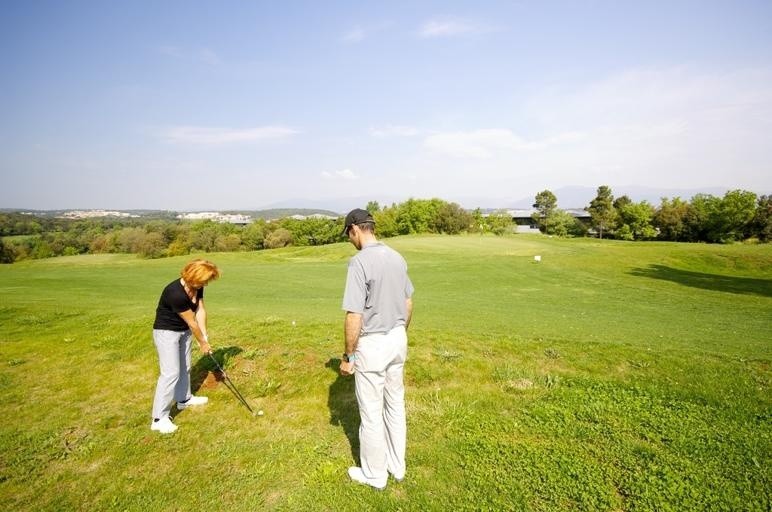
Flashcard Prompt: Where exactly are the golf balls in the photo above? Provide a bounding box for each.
[258,410,264,416]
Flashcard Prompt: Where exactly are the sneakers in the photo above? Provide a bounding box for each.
[150,414,179,434]
[177,394,209,410]
[348,466,389,490]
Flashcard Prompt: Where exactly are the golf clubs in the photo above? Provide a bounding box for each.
[209,350,258,417]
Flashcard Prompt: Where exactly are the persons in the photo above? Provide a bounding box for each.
[149,258,221,434]
[339,208,415,489]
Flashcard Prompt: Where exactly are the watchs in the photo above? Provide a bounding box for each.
[342,353,355,362]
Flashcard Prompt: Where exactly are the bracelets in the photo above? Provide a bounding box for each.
[204,335,209,341]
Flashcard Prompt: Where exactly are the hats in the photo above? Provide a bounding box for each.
[340,208,375,238]
